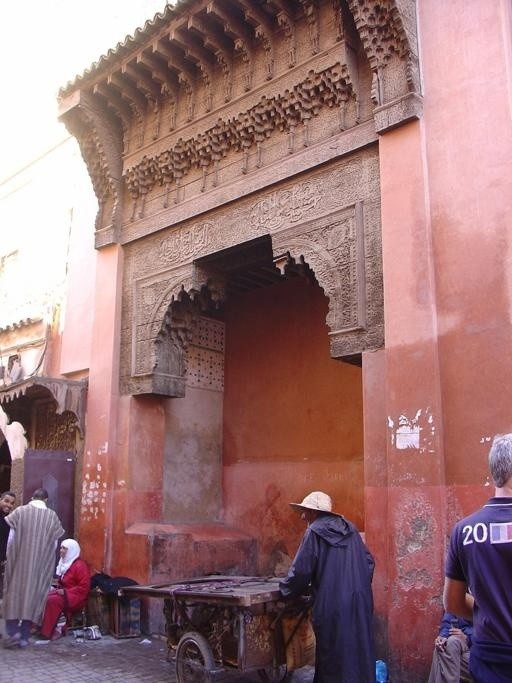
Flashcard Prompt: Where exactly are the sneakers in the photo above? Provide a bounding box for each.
[3,631,21,644]
[19,639,28,646]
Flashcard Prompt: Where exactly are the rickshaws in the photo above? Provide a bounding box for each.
[117,571,316,682]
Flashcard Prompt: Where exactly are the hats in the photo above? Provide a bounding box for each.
[287,490,345,517]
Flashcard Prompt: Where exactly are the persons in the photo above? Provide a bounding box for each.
[1,490,16,574]
[427,585,473,683]
[3,487,66,649]
[443,431,512,683]
[32,538,91,642]
[279,490,376,682]
